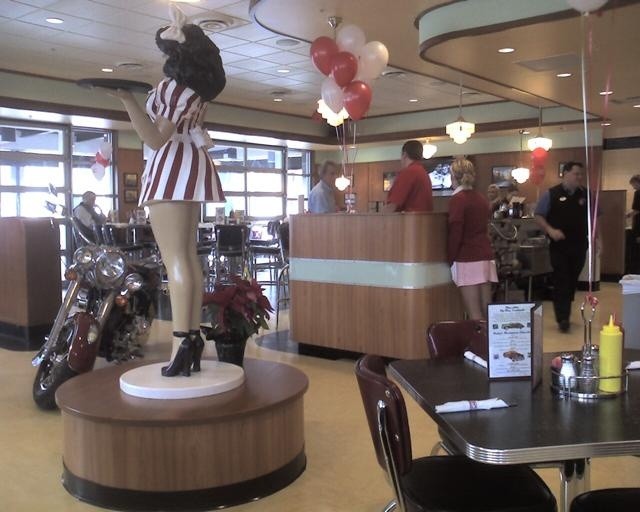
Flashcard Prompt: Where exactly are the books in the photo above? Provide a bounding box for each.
[487,304,543,391]
[509,196,528,209]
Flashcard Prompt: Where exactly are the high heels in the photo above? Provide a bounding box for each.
[161,329,204,377]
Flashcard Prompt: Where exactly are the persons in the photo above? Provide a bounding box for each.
[624,174,640,274]
[535,161,589,333]
[307,160,339,214]
[488,183,500,211]
[446,158,500,320]
[501,183,517,205]
[72,192,106,248]
[92,2,227,377]
[381,140,432,212]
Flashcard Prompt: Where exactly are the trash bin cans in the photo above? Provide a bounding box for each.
[618,274,640,350]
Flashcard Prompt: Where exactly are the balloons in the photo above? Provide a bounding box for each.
[310,25,390,123]
[95,151,111,167]
[99,141,113,160]
[90,163,106,180]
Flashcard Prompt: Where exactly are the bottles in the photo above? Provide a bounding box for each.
[599,312,626,392]
[230,209,234,219]
[559,351,578,389]
[579,355,598,392]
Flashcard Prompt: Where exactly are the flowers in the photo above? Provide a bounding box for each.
[197,274,273,341]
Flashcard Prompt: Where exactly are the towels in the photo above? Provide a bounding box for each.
[623,357,640,370]
[463,348,489,369]
[431,394,515,415]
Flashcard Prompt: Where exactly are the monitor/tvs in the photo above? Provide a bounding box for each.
[420,157,457,190]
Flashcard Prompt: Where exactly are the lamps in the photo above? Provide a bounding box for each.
[528,102,555,156]
[332,167,352,195]
[419,139,438,163]
[317,95,348,127]
[511,165,528,183]
[444,81,477,149]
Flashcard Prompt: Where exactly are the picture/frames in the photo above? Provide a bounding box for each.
[381,170,402,191]
[124,189,138,203]
[123,172,139,188]
[491,164,519,188]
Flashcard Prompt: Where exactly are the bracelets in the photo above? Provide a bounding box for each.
[547,228,555,236]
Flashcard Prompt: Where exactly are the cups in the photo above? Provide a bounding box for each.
[136,210,147,225]
[494,211,503,220]
[110,209,118,222]
[512,202,520,218]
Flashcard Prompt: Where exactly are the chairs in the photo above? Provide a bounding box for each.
[50,206,292,344]
[430,317,496,362]
[356,353,560,511]
[567,484,640,512]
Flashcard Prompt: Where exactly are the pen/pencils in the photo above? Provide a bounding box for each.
[532,377,542,393]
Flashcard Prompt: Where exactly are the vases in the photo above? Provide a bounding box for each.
[213,339,247,368]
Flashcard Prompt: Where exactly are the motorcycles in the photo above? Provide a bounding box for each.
[30,181,161,416]
